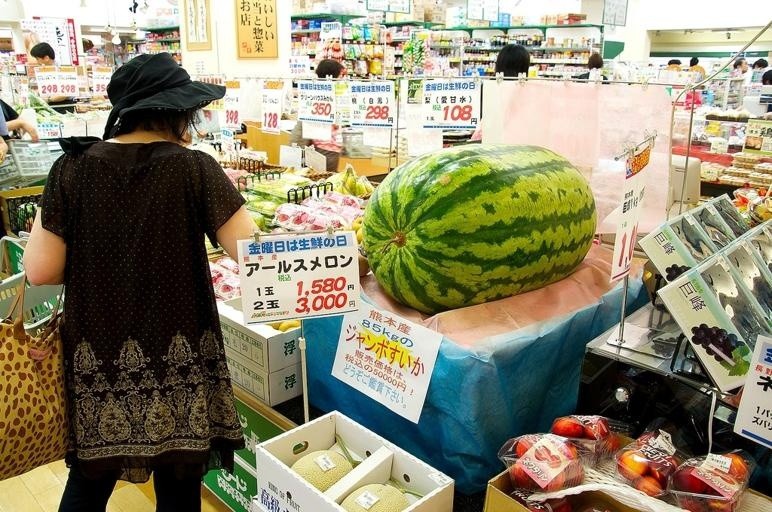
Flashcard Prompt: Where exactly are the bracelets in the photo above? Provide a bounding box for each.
[48,96,51,103]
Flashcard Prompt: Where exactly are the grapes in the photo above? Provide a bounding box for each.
[667,264,691,281]
[691,324,745,362]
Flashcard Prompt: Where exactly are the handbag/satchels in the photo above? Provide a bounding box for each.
[0,272,70,481]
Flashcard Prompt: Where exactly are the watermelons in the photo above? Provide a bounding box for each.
[361,143,598,315]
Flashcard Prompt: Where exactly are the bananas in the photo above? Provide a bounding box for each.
[334,166,374,197]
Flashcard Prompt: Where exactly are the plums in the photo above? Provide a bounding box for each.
[510,415,749,512]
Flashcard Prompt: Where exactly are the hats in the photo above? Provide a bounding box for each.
[101,52,227,139]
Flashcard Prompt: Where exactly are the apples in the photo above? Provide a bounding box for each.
[352,217,362,231]
[357,228,362,244]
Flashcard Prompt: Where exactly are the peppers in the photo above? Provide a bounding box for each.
[16,202,38,229]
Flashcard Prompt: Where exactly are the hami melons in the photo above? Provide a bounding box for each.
[340,479,424,512]
[290,433,360,493]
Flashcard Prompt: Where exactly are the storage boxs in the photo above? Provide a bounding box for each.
[216,298,772,512]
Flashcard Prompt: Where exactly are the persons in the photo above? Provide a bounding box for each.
[761,70,771,85]
[730,58,748,76]
[29,42,77,114]
[751,59,768,71]
[575,54,609,84]
[315,59,345,81]
[471,44,530,141]
[19,49,268,511]
[0,100,39,166]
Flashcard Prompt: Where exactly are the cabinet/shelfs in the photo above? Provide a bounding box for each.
[291,11,604,103]
